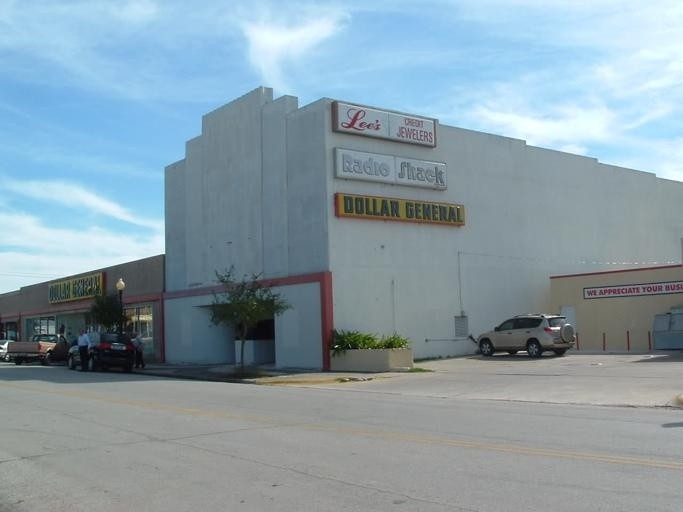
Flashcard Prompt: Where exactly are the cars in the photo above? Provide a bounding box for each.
[0,338,17,363]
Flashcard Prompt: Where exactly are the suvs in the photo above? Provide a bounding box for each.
[65,330,136,371]
[475,310,575,363]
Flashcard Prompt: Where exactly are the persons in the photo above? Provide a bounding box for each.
[133,334,146,370]
[78,329,91,372]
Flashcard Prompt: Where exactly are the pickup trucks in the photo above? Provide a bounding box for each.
[5,333,69,366]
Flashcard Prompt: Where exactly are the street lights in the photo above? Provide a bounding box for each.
[114,277,126,333]
